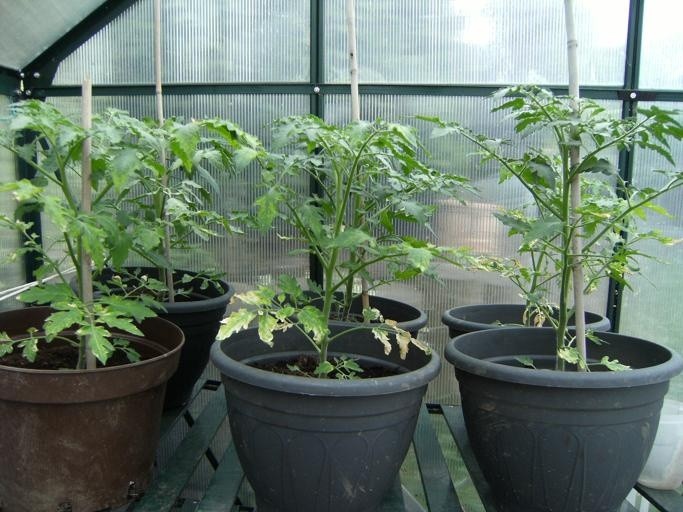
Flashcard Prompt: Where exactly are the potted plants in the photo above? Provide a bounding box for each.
[441,93,682,512]
[211,109,441,508]
[0,92,235,512]
